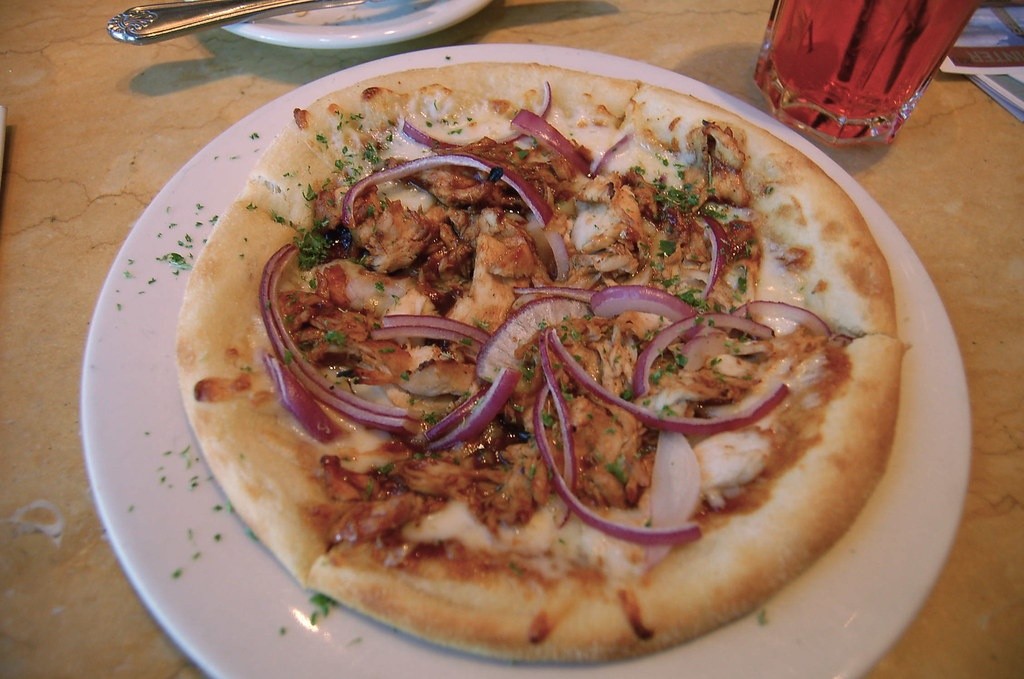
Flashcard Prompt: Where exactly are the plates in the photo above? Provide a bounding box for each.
[80,42,973,679]
[185,0,495,50]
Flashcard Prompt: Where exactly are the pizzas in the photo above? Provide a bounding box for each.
[176,68,904,668]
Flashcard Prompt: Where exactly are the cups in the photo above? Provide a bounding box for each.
[753,0,982,150]
